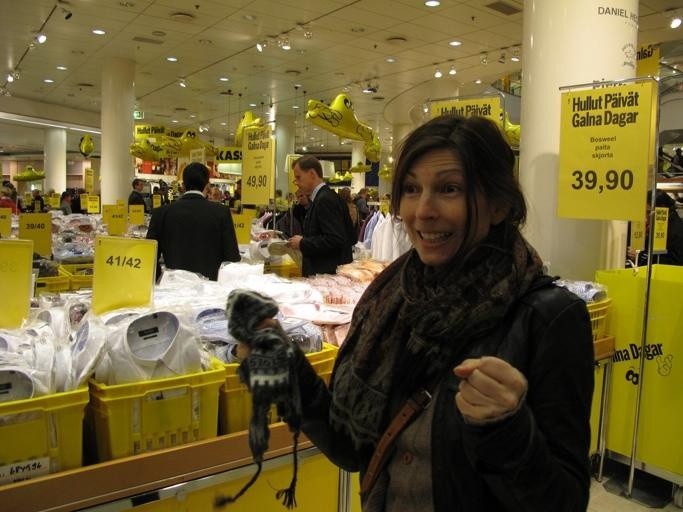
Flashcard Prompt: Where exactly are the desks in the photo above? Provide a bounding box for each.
[0,433,364,512]
[588,358,613,482]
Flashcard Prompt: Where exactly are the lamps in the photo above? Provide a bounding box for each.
[58,8,73,20]
[511,49,520,62]
[6,67,21,83]
[207,88,342,151]
[431,67,442,79]
[481,56,487,65]
[449,65,455,75]
[497,54,505,64]
[363,82,378,94]
[33,32,45,43]
[4,86,12,97]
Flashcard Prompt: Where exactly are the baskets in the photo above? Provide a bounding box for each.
[0,381,91,485]
[88,355,226,462]
[216,337,339,434]
[587,295,612,341]
[35,262,93,296]
[265,261,302,277]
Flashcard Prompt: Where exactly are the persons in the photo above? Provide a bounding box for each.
[141,192,151,212]
[222,191,232,203]
[151,186,163,208]
[26,187,45,212]
[625,191,683,268]
[381,192,390,200]
[338,186,357,227]
[227,106,593,512]
[2,180,17,201]
[274,189,282,199]
[352,187,370,220]
[282,153,357,279]
[227,178,241,207]
[158,178,170,201]
[143,160,253,286]
[290,187,312,237]
[71,187,86,214]
[60,190,73,216]
[0,186,21,214]
[670,148,683,175]
[286,193,293,204]
[128,178,148,213]
